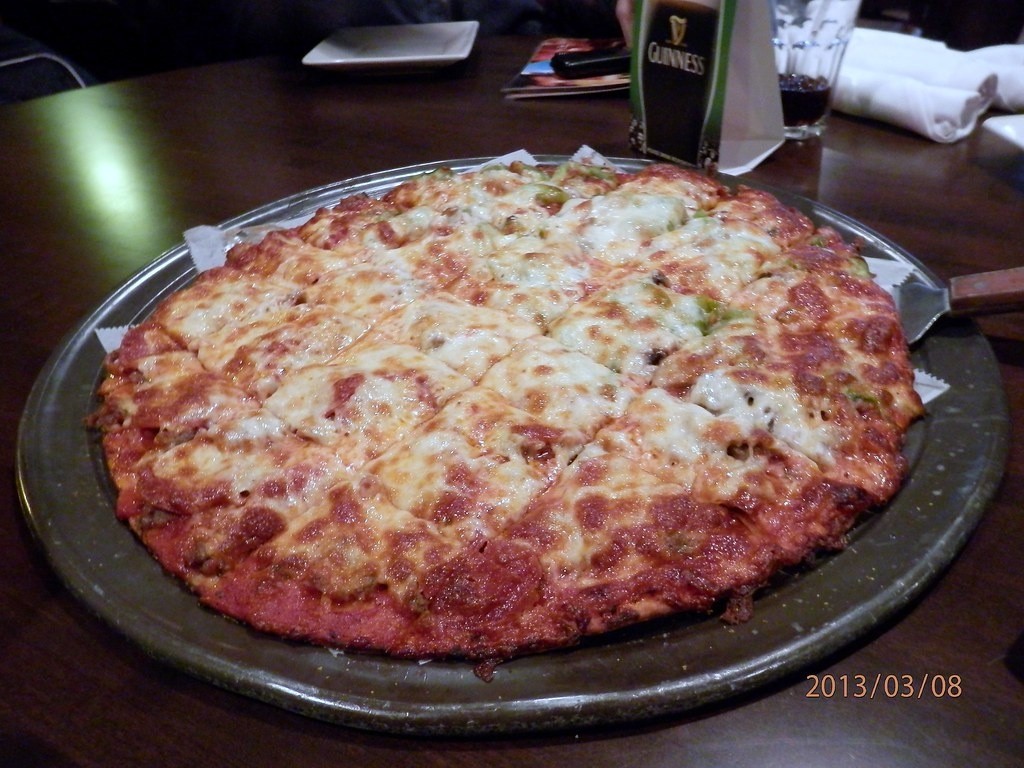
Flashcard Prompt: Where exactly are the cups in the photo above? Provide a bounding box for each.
[769,0,861,142]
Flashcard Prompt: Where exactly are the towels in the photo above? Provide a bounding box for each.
[828,26,1024,144]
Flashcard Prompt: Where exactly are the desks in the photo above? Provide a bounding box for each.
[0,14,1024,768]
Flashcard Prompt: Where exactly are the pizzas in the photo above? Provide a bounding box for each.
[86,160,924,656]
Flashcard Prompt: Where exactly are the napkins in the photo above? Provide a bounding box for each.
[779,23,1023,143]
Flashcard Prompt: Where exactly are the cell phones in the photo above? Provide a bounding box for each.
[549,46,632,80]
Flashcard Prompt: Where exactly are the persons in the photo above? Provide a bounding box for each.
[0,0,634,105]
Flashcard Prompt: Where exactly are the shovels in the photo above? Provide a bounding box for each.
[867,257,1024,345]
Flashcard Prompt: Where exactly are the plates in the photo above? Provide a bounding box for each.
[301,21,479,65]
[17,153,1007,733]
[981,114,1024,153]
